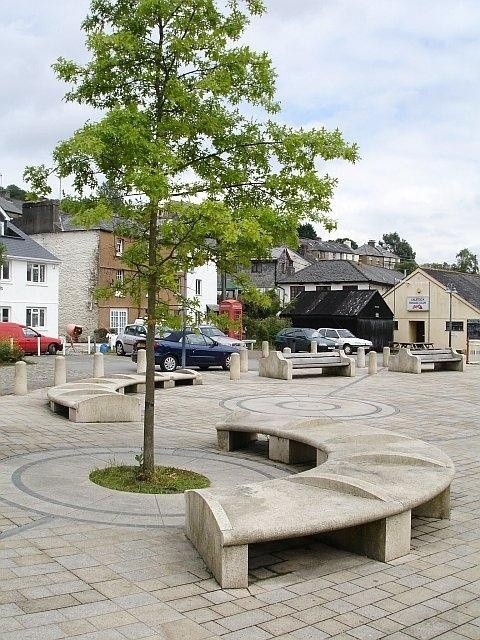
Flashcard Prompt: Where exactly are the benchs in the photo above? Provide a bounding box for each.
[388,347,465,374]
[185,411,456,589]
[47,368,202,423]
[389,341,434,351]
[259,350,356,381]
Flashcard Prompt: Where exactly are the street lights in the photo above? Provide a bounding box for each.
[445,283,458,347]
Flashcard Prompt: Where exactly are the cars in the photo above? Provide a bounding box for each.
[0,322,63,356]
[114,323,246,372]
[274,328,373,355]
